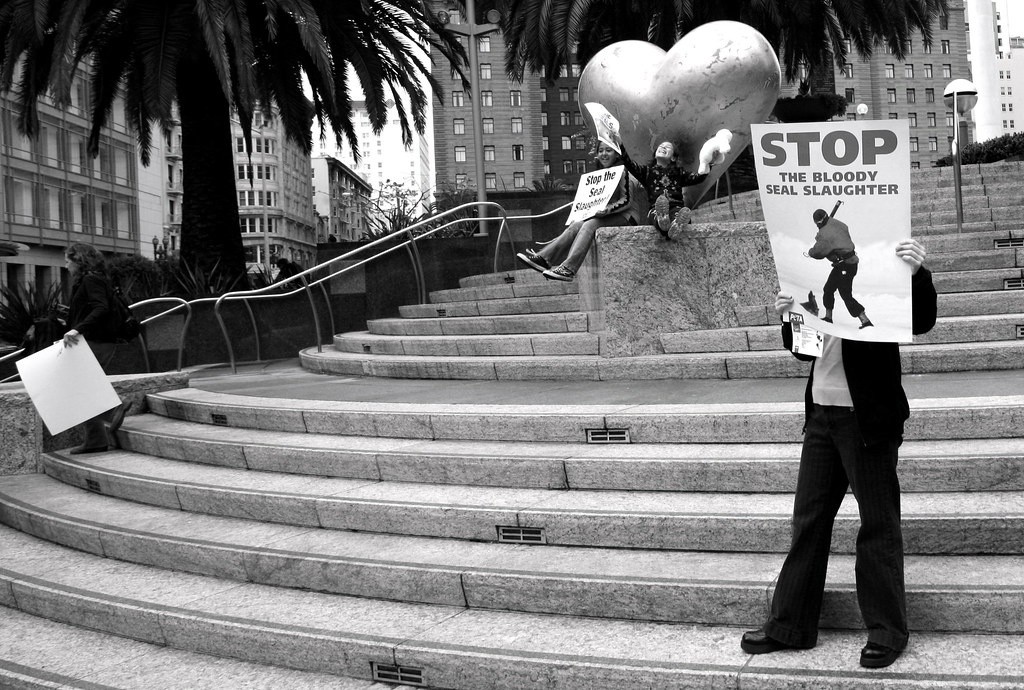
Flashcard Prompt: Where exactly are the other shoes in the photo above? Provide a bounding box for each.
[70,446,108,454]
[109,398,131,433]
[655,195,670,232]
[668,207,692,239]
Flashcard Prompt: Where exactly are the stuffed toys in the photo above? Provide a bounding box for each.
[698,128,732,175]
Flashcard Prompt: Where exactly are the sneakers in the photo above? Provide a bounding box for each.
[542,266,574,282]
[517,248,551,272]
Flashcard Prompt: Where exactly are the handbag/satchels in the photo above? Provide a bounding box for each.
[89,273,142,342]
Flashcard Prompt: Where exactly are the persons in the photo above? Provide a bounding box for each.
[274,258,292,283]
[741,239,938,668]
[809,209,873,329]
[59,242,133,455]
[517,141,640,282]
[614,132,719,239]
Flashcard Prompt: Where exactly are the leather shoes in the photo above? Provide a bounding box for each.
[741,628,791,654]
[860,640,901,668]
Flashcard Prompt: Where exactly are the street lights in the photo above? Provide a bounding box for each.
[857,103,868,120]
[942,78,978,232]
[437,8,501,237]
